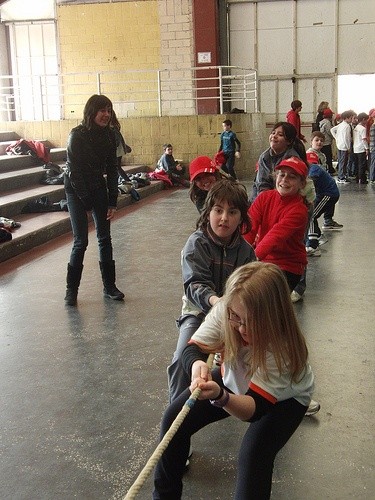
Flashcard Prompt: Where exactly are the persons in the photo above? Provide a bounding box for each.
[152,261,316,500]
[286,100,375,188]
[164,182,321,419]
[217,120,241,182]
[190,122,344,305]
[63,94,127,305]
[157,143,190,186]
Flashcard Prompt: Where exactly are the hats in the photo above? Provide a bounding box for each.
[291,101,302,109]
[323,109,335,117]
[274,155,308,178]
[188,155,217,182]
[306,152,319,164]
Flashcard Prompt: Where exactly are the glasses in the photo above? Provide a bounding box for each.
[227,308,250,336]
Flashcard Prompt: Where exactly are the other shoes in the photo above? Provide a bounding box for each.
[358,179,368,184]
[290,289,306,303]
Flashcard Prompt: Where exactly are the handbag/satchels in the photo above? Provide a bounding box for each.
[214,150,225,167]
[29,195,68,211]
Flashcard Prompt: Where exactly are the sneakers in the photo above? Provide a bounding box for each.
[305,245,321,257]
[184,445,194,467]
[317,232,329,246]
[322,220,344,231]
[304,399,320,415]
[337,178,352,184]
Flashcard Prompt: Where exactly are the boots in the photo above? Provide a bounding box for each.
[99,260,125,300]
[64,262,84,306]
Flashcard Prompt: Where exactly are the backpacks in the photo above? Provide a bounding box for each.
[118,171,151,193]
[39,169,65,185]
